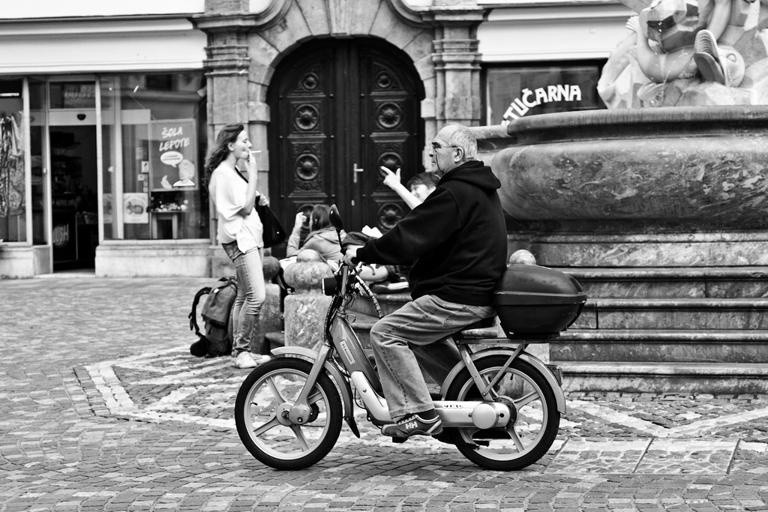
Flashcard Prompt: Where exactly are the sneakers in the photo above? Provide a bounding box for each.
[465,373,505,400]
[234,349,260,369]
[231,347,273,369]
[380,411,446,439]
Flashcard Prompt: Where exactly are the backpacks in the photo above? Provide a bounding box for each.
[200,273,240,356]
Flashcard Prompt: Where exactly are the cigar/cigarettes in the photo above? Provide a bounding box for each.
[251,150,262,154]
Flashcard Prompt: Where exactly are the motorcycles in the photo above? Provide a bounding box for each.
[231,202,584,473]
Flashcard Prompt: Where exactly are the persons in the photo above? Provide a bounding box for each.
[199,121,274,372]
[378,164,444,212]
[275,203,349,329]
[159,158,195,190]
[341,120,511,440]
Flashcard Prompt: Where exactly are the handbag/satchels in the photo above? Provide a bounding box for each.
[251,194,288,250]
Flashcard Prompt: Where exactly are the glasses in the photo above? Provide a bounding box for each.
[431,144,461,152]
